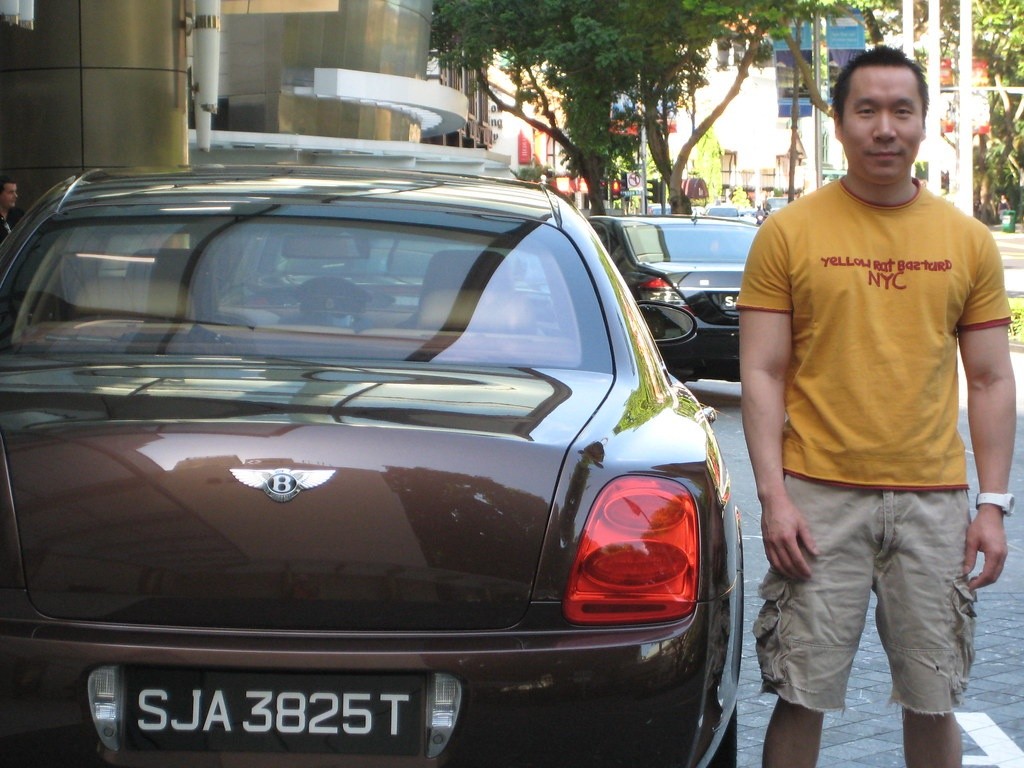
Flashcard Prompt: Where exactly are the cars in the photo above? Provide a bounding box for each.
[648,204,672,215]
[587,215,763,387]
[693,206,744,221]
[765,198,788,214]
[0,166,746,768]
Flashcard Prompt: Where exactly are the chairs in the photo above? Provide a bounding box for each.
[128,246,219,322]
[75,277,195,320]
[399,250,510,331]
[416,288,536,332]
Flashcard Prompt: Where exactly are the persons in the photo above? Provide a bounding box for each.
[756,204,765,225]
[0,175,24,244]
[998,193,1010,220]
[735,44,1016,768]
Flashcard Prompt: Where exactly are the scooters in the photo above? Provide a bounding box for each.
[755,215,763,224]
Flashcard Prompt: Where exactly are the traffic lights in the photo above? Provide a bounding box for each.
[645,178,658,203]
[619,172,627,194]
[611,178,619,197]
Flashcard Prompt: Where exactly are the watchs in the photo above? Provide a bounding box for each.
[976,492,1015,517]
